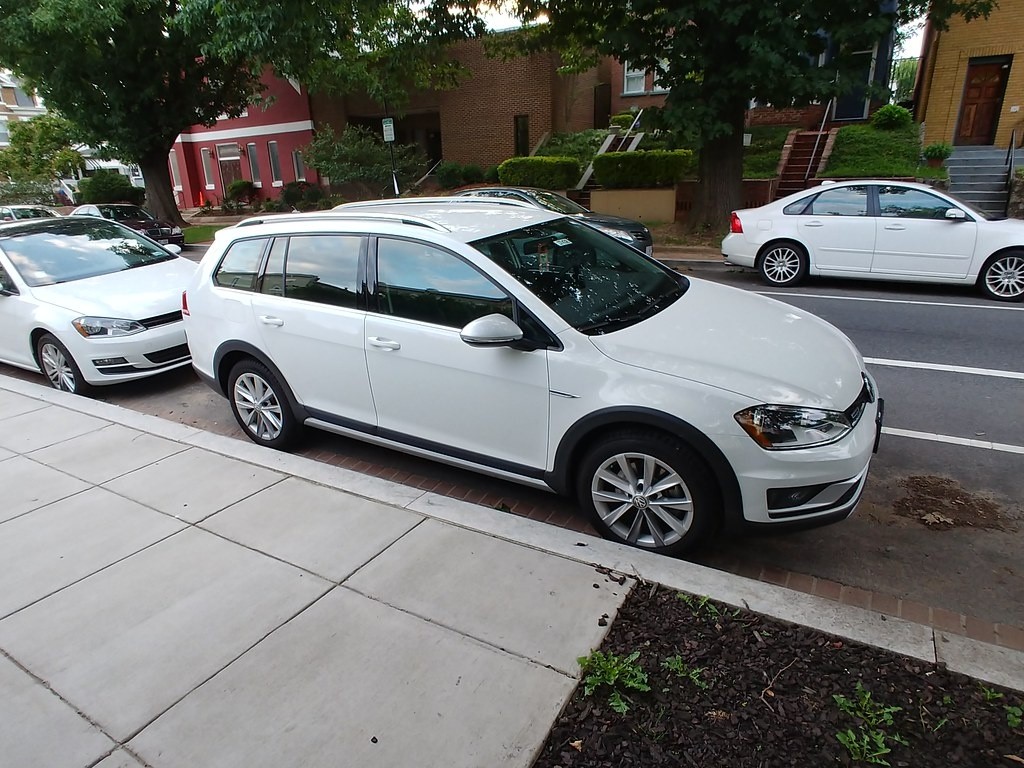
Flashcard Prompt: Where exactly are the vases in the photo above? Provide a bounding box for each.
[609,126,621,134]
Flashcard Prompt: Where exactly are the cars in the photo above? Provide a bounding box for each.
[0,216,201,394]
[69,203,185,251]
[721,177,1024,302]
[448,187,653,264]
[0,205,61,223]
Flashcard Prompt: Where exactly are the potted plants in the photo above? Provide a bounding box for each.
[923,142,955,167]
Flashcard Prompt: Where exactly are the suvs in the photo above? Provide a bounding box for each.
[180,197,883,557]
